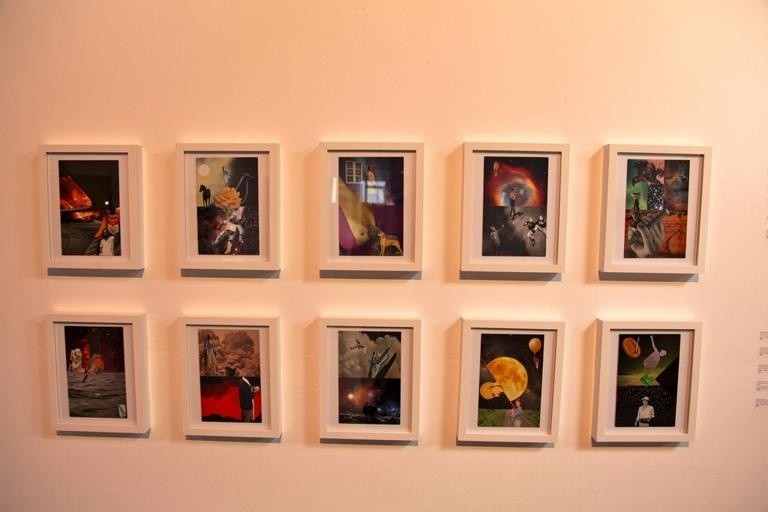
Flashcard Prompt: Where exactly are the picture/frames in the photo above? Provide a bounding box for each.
[457,315,566,445]
[318,143,424,274]
[591,317,703,445]
[177,142,283,272]
[600,145,712,274]
[182,314,284,441]
[43,313,152,436]
[40,143,146,273]
[462,142,566,274]
[318,315,422,442]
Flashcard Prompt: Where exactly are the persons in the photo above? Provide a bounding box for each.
[510,397,522,427]
[634,396,655,426]
[488,188,547,250]
[99,229,114,257]
[628,214,664,258]
[70,348,104,377]
[480,381,504,400]
[239,377,260,422]
